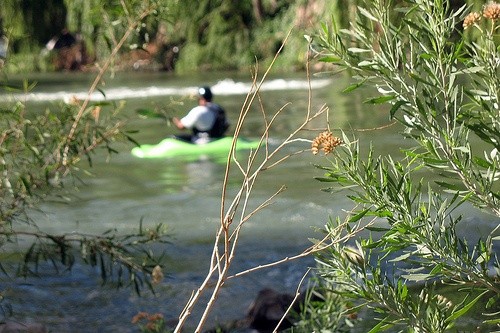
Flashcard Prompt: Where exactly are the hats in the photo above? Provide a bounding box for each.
[194,87,211,98]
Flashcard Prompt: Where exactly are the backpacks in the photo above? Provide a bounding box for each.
[198,104,228,137]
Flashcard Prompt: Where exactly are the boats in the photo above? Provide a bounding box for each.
[129,136,269,159]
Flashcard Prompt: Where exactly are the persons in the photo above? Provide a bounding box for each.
[169,84,231,145]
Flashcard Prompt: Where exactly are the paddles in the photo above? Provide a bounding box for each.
[134,109,233,136]
